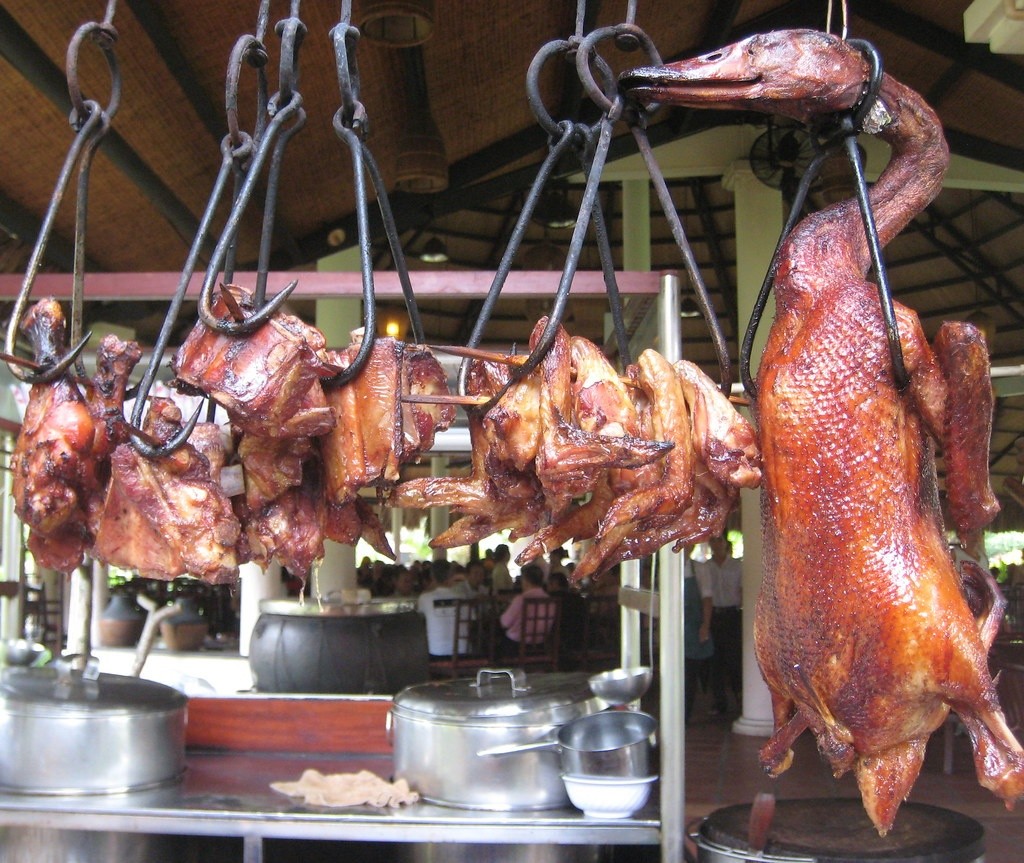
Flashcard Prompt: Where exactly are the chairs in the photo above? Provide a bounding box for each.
[427,591,623,679]
[16,574,236,646]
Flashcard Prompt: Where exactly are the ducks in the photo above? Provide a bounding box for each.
[613,23,1024,839]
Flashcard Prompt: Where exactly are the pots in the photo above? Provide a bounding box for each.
[383,665,594,813]
[474,710,662,787]
[0,648,190,795]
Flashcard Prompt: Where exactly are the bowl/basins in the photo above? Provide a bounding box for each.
[559,772,658,818]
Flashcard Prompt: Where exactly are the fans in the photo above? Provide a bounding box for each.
[750,125,823,195]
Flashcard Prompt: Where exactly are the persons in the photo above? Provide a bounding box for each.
[951,525,1024,634]
[281,525,744,725]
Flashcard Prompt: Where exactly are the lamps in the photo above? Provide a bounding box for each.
[418,236,448,264]
[544,196,580,229]
